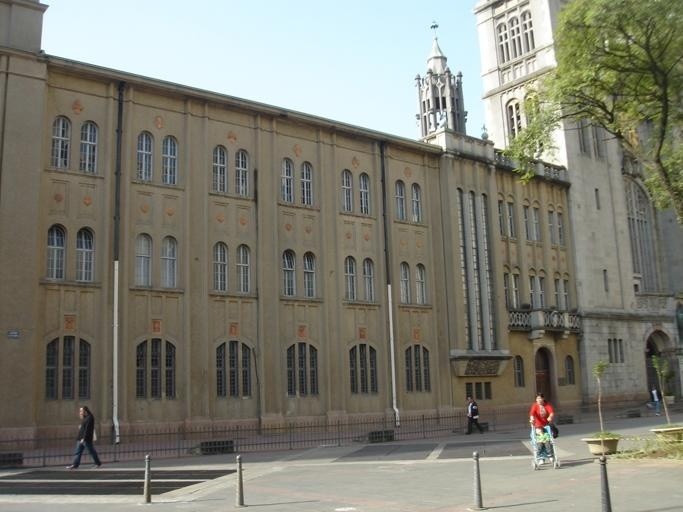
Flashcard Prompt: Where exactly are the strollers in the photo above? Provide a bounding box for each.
[529,421,560,469]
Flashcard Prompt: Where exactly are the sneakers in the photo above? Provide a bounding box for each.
[66,464,103,469]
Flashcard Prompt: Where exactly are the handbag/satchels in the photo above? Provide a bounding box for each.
[550,424,559,438]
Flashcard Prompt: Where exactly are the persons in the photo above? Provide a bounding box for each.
[649,384,662,416]
[66,405,103,470]
[528,391,555,465]
[535,427,553,457]
[465,395,484,434]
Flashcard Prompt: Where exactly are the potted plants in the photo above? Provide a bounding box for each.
[582,361,619,454]
[651,355,683,442]
